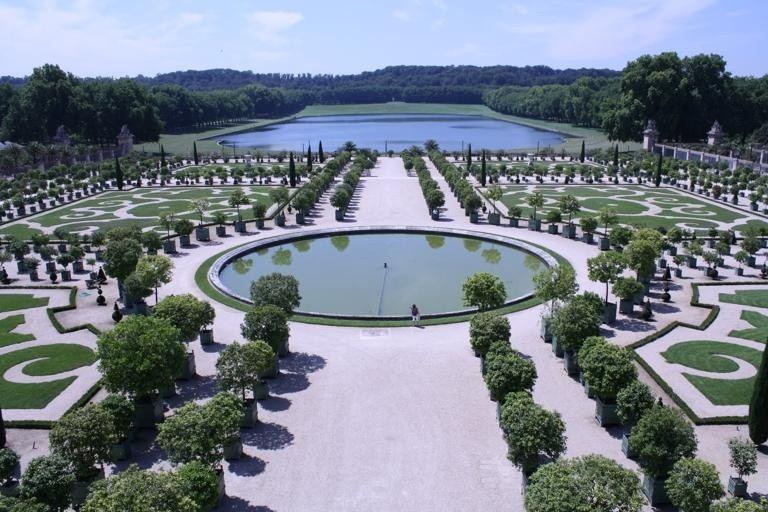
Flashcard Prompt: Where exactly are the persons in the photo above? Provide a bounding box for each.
[409,304,420,327]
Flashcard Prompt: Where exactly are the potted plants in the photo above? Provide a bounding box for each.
[458,254,767,512]
[2,140,399,178]
[1,178,364,272]
[0,273,306,511]
[398,137,767,255]
